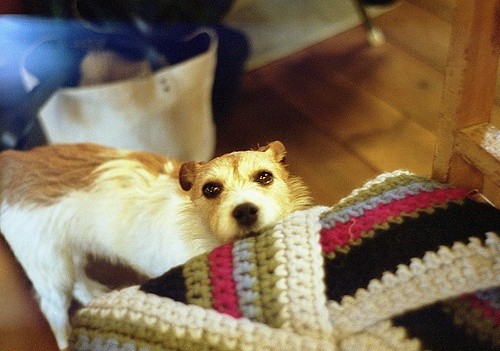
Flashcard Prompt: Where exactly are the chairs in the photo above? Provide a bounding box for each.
[68,0,499,351]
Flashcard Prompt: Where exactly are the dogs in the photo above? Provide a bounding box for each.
[0,141,317,348]
